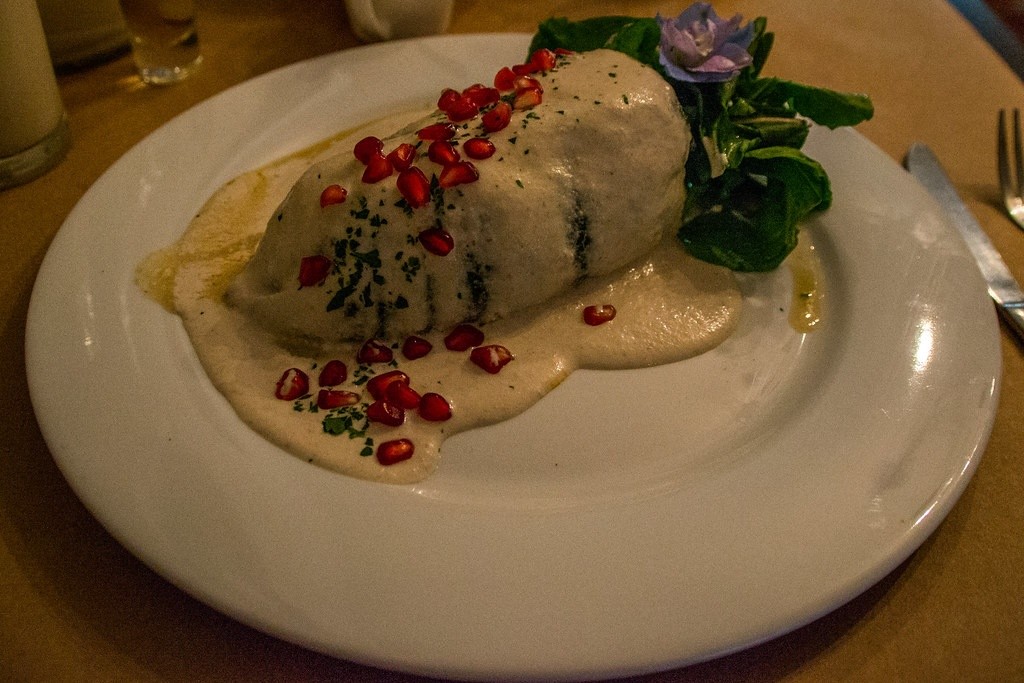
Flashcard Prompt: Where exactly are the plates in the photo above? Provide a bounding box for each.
[28,33,1000,683]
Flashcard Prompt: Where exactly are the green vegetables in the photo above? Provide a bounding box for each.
[528,13,876,272]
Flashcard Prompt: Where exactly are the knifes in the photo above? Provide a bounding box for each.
[907,142,1024,331]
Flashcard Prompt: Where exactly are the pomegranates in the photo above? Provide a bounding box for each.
[277,49,617,466]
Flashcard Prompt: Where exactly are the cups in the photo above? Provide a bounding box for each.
[0,0,71,192]
[350,0,453,42]
[117,0,201,88]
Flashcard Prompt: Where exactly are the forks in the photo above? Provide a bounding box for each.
[997,107,1024,228]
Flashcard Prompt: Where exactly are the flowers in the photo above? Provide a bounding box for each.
[657,0,755,83]
